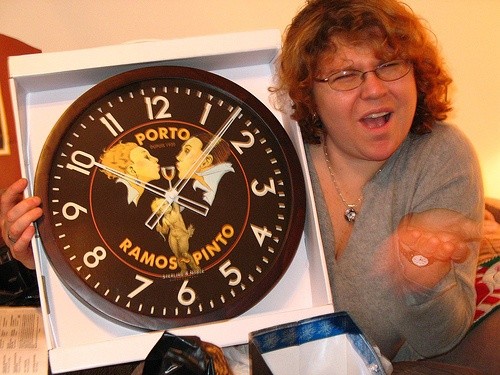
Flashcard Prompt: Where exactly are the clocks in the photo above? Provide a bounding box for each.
[32,65,309,335]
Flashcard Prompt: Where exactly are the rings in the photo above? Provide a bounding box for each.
[7,231,17,244]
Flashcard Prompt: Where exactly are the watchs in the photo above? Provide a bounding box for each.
[398,240,437,268]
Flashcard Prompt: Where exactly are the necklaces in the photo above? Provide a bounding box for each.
[320,132,388,224]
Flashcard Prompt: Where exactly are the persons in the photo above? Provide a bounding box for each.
[0,0,486,363]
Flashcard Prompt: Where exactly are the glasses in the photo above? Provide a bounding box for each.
[312,58,413,92]
